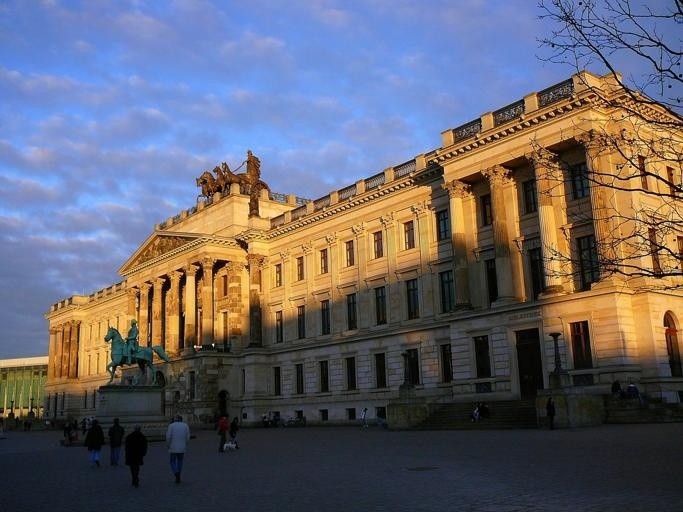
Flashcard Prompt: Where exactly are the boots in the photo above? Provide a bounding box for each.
[174,472,181,484]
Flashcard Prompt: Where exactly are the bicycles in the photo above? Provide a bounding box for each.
[357,418,368,432]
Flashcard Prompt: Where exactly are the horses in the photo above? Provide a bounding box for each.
[104,326,171,387]
[196,162,241,198]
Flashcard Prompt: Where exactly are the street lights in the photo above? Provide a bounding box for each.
[549,332,565,374]
[11,400,14,413]
[30,398,34,411]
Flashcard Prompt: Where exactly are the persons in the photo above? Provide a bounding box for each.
[107,418,125,468]
[358,407,370,433]
[242,149,261,179]
[627,382,639,399]
[610,378,625,403]
[546,397,557,430]
[469,402,491,423]
[84,419,105,469]
[15,415,32,431]
[123,317,140,367]
[216,412,230,454]
[165,415,191,485]
[123,424,148,489]
[228,416,243,450]
[63,418,92,448]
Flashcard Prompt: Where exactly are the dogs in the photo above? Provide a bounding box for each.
[222,440,241,452]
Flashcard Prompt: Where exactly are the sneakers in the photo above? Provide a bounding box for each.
[132,478,140,489]
[95,460,100,467]
[218,448,224,453]
